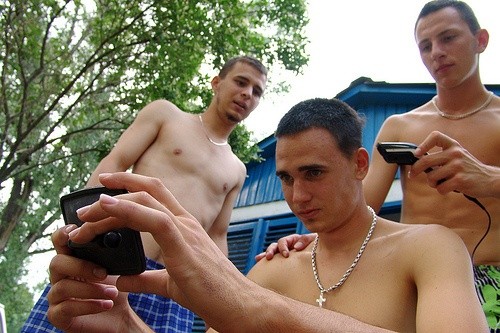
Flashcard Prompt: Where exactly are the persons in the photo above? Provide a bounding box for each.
[21,55,269,333]
[256,0,500,333]
[46,97,489,333]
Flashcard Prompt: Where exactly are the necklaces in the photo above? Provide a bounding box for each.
[199,114,228,146]
[432,91,493,120]
[311,205,377,308]
